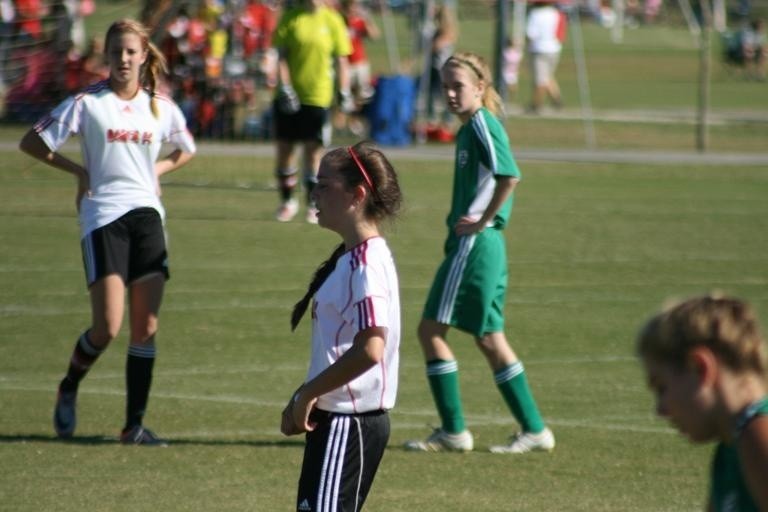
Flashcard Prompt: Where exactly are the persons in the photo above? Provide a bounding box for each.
[407,1,569,142]
[1,0,383,131]
[16,19,199,448]
[636,289,765,509]
[271,1,356,223]
[593,1,665,32]
[275,142,401,511]
[404,51,556,456]
[725,9,766,78]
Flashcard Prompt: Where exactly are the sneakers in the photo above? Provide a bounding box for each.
[53,379,77,436]
[307,206,320,223]
[121,425,164,445]
[404,427,472,452]
[490,426,556,456]
[276,202,298,221]
